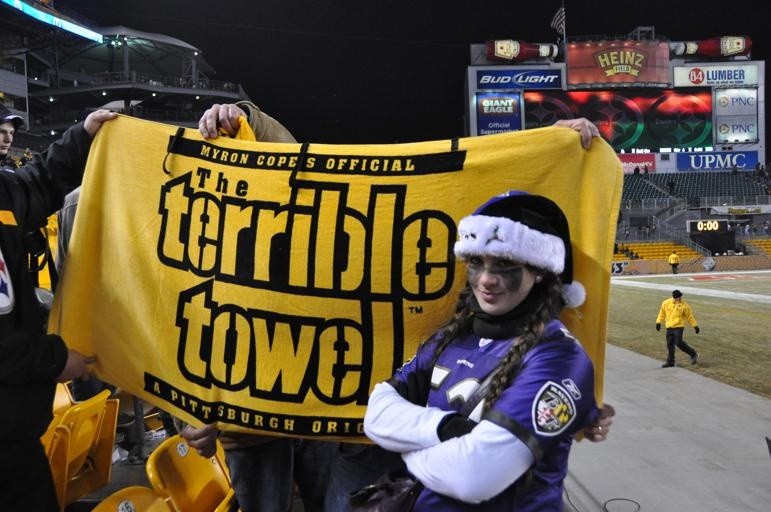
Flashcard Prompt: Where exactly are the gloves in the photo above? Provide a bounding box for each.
[397,368,429,406]
[438,413,473,441]
[694,327,699,334]
[656,324,660,331]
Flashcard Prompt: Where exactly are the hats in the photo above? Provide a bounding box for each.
[453,195,586,309]
[0,103,24,125]
[673,290,682,298]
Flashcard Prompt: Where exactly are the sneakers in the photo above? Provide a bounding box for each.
[117,413,136,426]
[691,352,699,365]
[662,363,675,367]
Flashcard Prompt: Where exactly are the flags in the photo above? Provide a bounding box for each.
[43,110,627,446]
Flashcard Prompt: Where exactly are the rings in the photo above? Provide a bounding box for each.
[599,425,604,432]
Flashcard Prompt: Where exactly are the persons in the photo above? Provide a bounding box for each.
[0,104,25,166]
[170,96,340,509]
[755,162,771,184]
[644,165,649,174]
[656,290,701,368]
[53,185,117,402]
[668,250,680,274]
[0,106,122,512]
[634,165,641,176]
[727,220,771,238]
[19,149,33,167]
[324,119,619,512]
[669,178,676,195]
[733,164,738,173]
[362,194,599,512]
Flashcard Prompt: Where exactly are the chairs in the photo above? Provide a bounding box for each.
[611,236,771,262]
[618,170,768,205]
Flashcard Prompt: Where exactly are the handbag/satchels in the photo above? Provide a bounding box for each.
[347,470,424,512]
